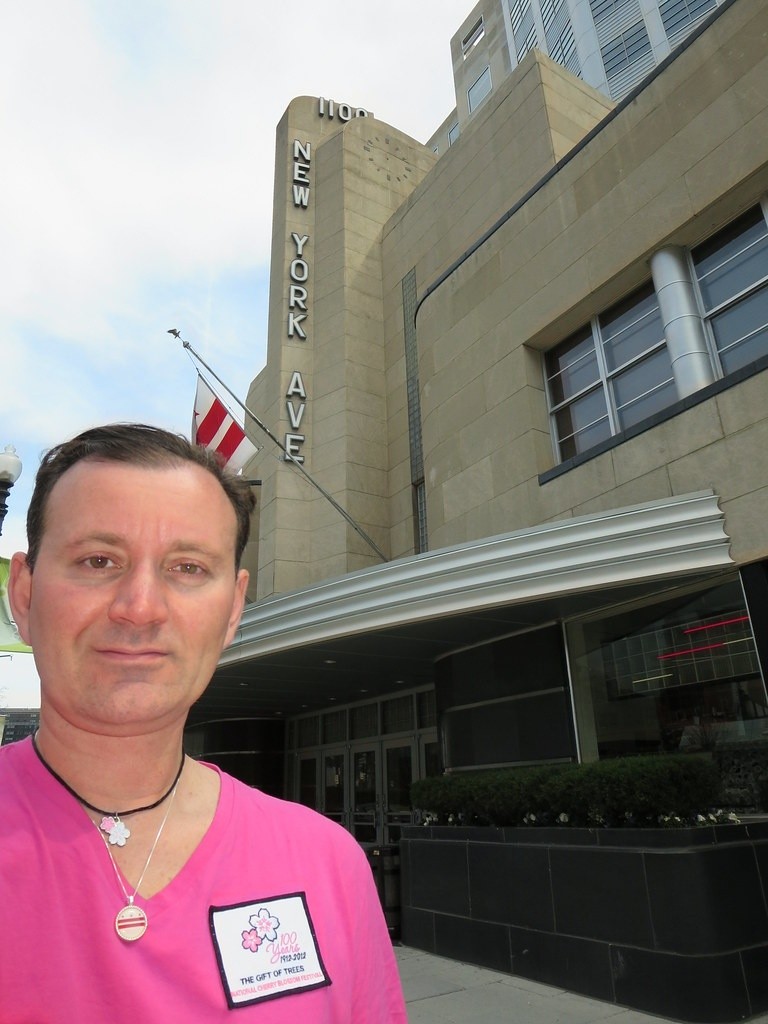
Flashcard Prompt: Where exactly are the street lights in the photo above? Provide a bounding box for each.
[0,444,24,535]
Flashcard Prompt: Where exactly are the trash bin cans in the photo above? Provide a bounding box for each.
[365,845,403,936]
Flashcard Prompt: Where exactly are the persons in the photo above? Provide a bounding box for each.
[0,423,407,1024]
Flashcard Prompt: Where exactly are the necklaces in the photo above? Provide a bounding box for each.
[31,723,186,941]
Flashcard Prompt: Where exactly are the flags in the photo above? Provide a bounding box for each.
[190,371,259,476]
